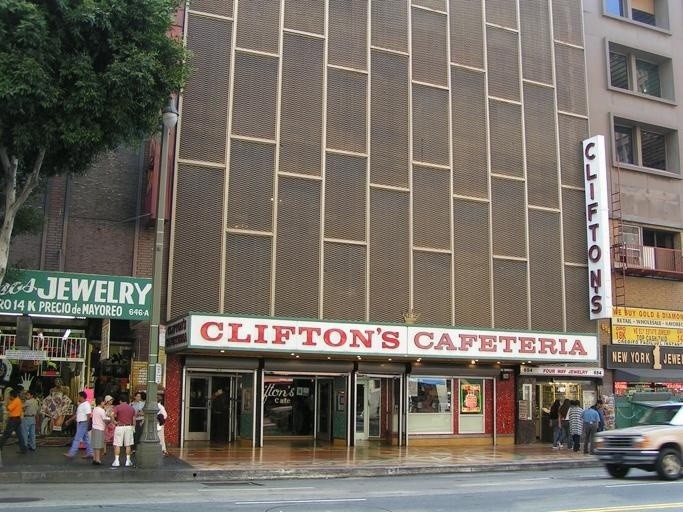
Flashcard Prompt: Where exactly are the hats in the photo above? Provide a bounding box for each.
[104,395,114,404]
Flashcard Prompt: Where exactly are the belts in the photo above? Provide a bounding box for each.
[119,424,133,426]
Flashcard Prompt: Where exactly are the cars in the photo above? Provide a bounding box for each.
[358,405,380,421]
[268,405,294,427]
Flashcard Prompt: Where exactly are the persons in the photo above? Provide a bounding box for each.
[547,398,606,456]
[0,384,169,467]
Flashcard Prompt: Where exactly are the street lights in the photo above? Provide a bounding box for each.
[131,97,179,473]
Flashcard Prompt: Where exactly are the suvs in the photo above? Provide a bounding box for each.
[589,401,682,479]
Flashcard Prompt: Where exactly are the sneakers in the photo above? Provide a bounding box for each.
[112,460,120,467]
[125,460,133,466]
